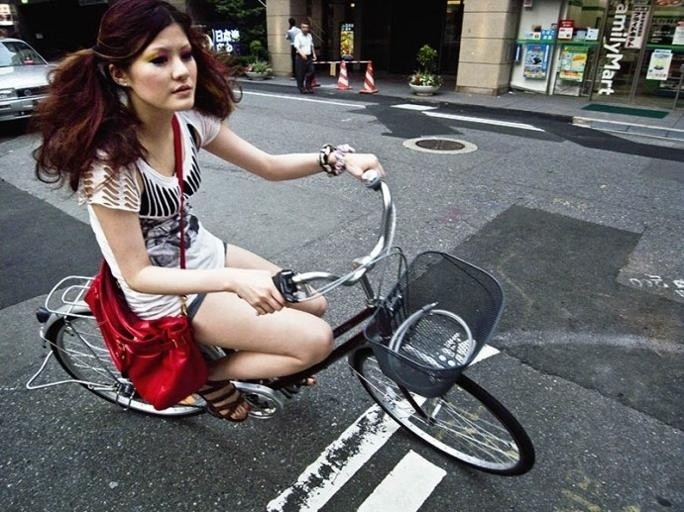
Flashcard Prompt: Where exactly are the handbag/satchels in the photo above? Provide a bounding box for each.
[84,258,210,411]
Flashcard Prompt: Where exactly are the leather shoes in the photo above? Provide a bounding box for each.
[300,87,315,94]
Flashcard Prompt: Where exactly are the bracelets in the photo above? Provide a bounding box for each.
[333,143,354,175]
[319,142,335,176]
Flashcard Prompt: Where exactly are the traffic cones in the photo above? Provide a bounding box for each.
[359,60,378,94]
[336,59,353,90]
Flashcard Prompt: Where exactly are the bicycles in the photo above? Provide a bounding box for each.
[24,164,535,477]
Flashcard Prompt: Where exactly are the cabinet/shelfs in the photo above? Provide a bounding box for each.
[604,13,684,97]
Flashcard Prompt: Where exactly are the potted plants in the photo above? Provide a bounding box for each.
[243,39,273,82]
[407,44,444,97]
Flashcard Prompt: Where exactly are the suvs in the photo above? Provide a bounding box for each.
[0,36,63,126]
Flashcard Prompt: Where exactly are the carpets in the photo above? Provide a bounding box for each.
[581,103,669,119]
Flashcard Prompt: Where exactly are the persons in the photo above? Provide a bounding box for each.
[31,0,389,423]
[291,21,317,95]
[282,18,303,81]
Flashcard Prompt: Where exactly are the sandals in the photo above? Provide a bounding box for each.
[198,379,251,424]
